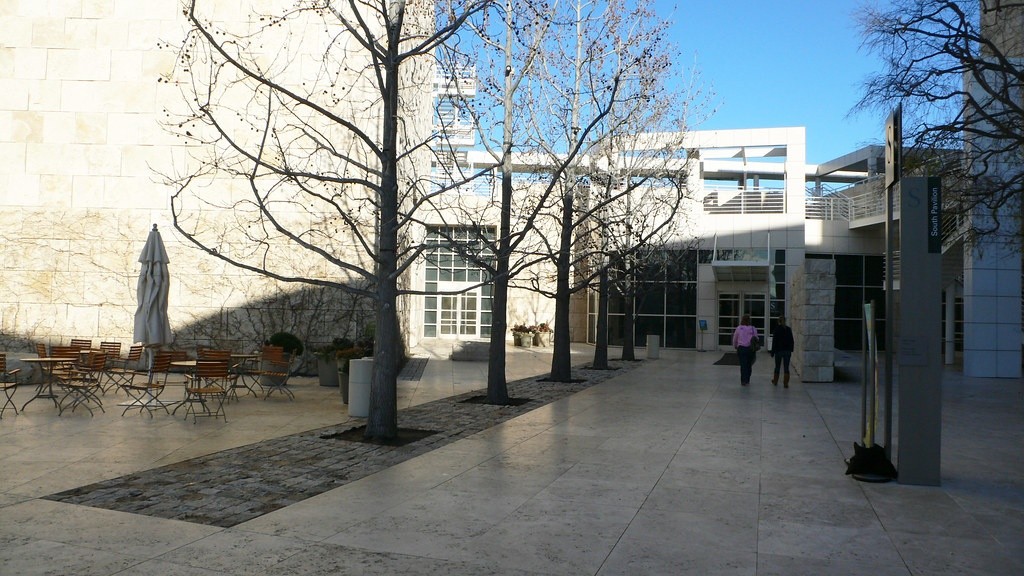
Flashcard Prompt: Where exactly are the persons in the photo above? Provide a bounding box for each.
[769,315,795,388]
[732,313,760,387]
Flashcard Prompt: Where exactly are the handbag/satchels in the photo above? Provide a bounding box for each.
[750,336,761,352]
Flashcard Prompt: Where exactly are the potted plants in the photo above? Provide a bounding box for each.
[313,332,375,406]
[511,321,554,349]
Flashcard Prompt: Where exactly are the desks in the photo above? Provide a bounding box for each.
[170,358,225,414]
[229,354,264,399]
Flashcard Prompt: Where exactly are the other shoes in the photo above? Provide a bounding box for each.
[741,382,749,385]
[772,380,789,388]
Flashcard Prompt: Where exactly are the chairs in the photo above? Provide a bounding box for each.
[20,338,190,419]
[263,346,295,401]
[194,347,232,397]
[0,353,20,418]
[254,348,284,395]
[180,358,230,426]
